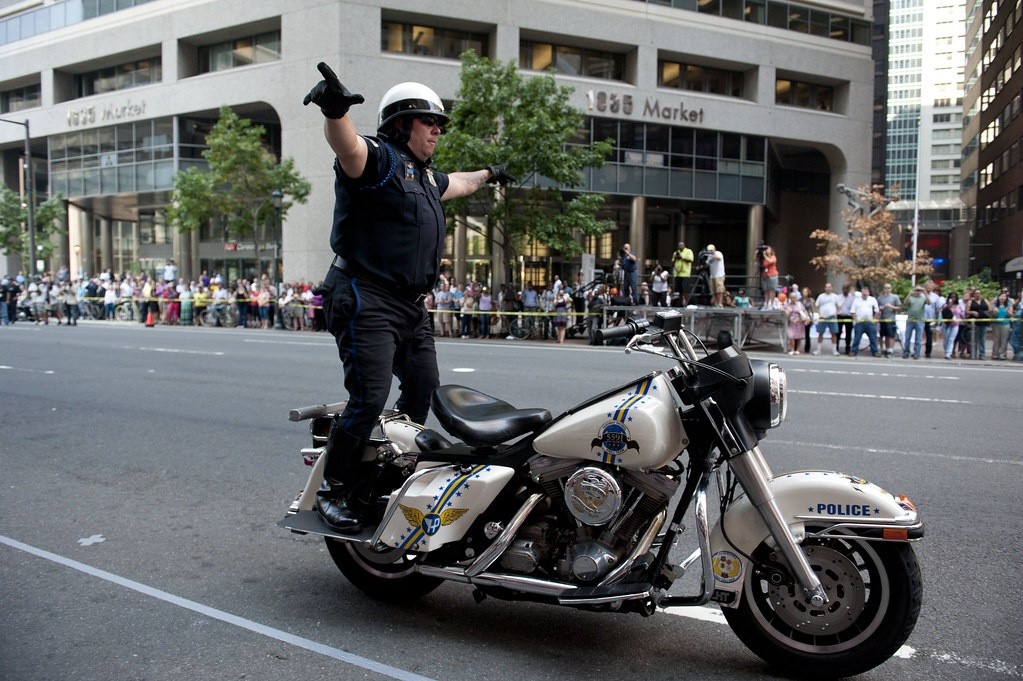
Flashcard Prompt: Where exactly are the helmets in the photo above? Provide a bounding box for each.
[378,82,450,132]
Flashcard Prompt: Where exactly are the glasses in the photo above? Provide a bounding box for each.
[1001,291,1009,293]
[400,113,446,128]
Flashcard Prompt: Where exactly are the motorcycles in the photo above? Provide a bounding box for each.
[275,306,930,680]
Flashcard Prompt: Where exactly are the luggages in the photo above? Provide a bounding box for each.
[507,335,516,340]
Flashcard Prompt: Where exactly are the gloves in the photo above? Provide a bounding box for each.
[484,162,517,186]
[304,63,364,119]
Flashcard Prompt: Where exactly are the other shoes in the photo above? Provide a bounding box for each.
[462,335,470,339]
[830,351,840,356]
[886,352,892,358]
[812,350,821,356]
[913,354,918,359]
[555,340,565,346]
[944,354,1009,361]
[923,352,933,359]
[788,350,800,355]
[903,352,909,358]
[66,322,71,325]
[848,352,856,357]
[674,301,773,311]
[873,351,880,358]
[478,336,490,339]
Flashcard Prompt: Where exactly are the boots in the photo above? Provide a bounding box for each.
[316,413,371,534]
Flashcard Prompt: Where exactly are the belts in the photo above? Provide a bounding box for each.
[333,255,428,306]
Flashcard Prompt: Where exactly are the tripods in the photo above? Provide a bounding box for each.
[684,271,710,308]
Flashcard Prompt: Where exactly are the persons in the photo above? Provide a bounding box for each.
[304,63,516,533]
[0,243,1023,362]
